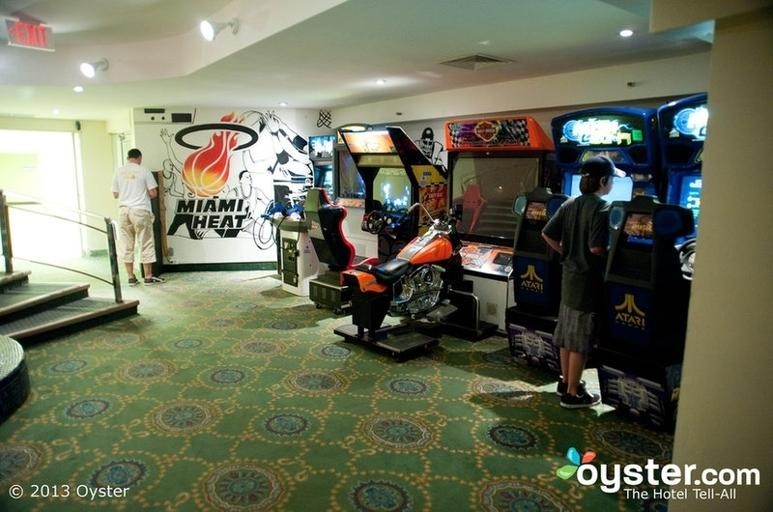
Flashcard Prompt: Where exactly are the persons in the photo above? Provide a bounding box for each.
[112,149,166,287]
[223,171,273,238]
[159,157,184,199]
[542,153,616,409]
[261,109,313,187]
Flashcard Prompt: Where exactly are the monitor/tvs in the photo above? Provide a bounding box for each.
[673,175,703,247]
[372,166,412,212]
[317,170,333,188]
[571,173,633,205]
[449,152,541,248]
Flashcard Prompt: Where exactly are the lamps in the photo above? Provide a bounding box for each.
[81,58,109,78]
[200,16,240,41]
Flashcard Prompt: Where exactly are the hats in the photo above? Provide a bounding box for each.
[579,154,623,178]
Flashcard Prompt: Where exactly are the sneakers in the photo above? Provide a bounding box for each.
[143,273,167,286]
[127,274,140,286]
[556,376,586,396]
[559,382,602,410]
